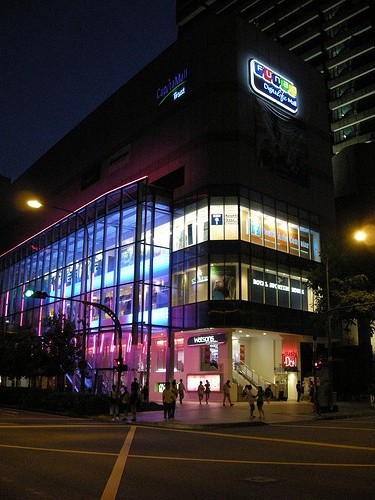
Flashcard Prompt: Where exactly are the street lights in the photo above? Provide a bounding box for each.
[27,200,90,394]
[325,230,367,391]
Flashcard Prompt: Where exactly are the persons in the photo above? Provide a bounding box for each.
[296,380,302,402]
[265,385,275,398]
[242,384,248,396]
[178,379,185,405]
[246,385,256,418]
[198,381,210,404]
[222,380,234,406]
[308,381,314,402]
[312,392,320,415]
[255,386,269,420]
[162,381,178,420]
[109,377,149,422]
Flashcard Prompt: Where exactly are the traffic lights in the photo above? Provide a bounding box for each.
[314,359,322,369]
[24,290,47,299]
[114,358,128,373]
